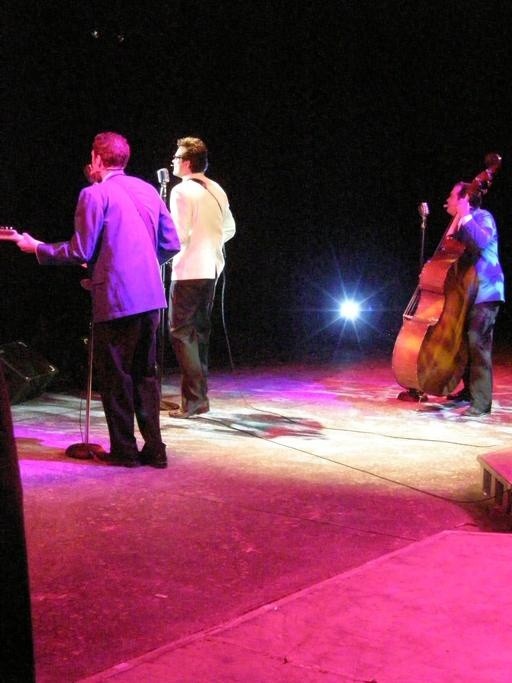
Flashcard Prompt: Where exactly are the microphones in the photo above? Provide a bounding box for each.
[84,164,100,184]
[157,168,170,186]
[420,200,430,217]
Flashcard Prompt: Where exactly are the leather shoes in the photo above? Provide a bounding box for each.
[168,402,209,419]
[463,405,490,416]
[98,451,167,468]
[448,389,471,401]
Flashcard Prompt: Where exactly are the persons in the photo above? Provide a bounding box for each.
[417,179,508,416]
[166,135,239,420]
[15,129,183,472]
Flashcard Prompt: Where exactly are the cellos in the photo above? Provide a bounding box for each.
[392,153,501,396]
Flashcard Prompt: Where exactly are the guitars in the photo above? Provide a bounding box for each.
[0,225,96,294]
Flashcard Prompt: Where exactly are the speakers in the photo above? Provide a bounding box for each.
[0,339,59,405]
